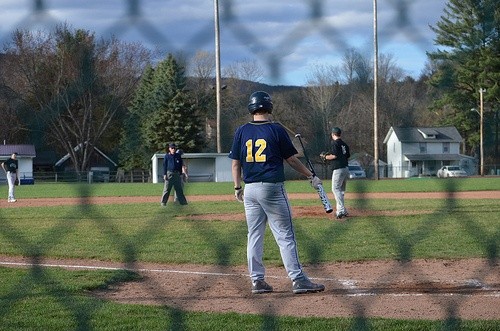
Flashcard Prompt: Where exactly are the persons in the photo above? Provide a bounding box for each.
[174,149,188,202]
[1,152,18,202]
[161,143,188,207]
[319,127,351,219]
[228,92,325,294]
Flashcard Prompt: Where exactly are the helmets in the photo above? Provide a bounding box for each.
[248,91,273,114]
[176,149,183,155]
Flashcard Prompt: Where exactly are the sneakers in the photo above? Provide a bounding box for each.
[252,280,274,294]
[292,277,325,293]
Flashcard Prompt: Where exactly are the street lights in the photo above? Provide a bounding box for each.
[470,87,487,176]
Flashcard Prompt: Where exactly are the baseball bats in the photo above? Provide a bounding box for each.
[295,132,334,214]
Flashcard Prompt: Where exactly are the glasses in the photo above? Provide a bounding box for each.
[172,148,175,149]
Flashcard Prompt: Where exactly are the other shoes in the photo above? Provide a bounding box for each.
[336,211,348,218]
[8,198,17,202]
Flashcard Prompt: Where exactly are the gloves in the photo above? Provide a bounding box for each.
[234,186,244,202]
[307,173,321,188]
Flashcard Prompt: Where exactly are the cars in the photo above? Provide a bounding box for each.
[437,165,468,178]
[347,164,366,180]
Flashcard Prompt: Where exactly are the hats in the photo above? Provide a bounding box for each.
[169,144,176,148]
